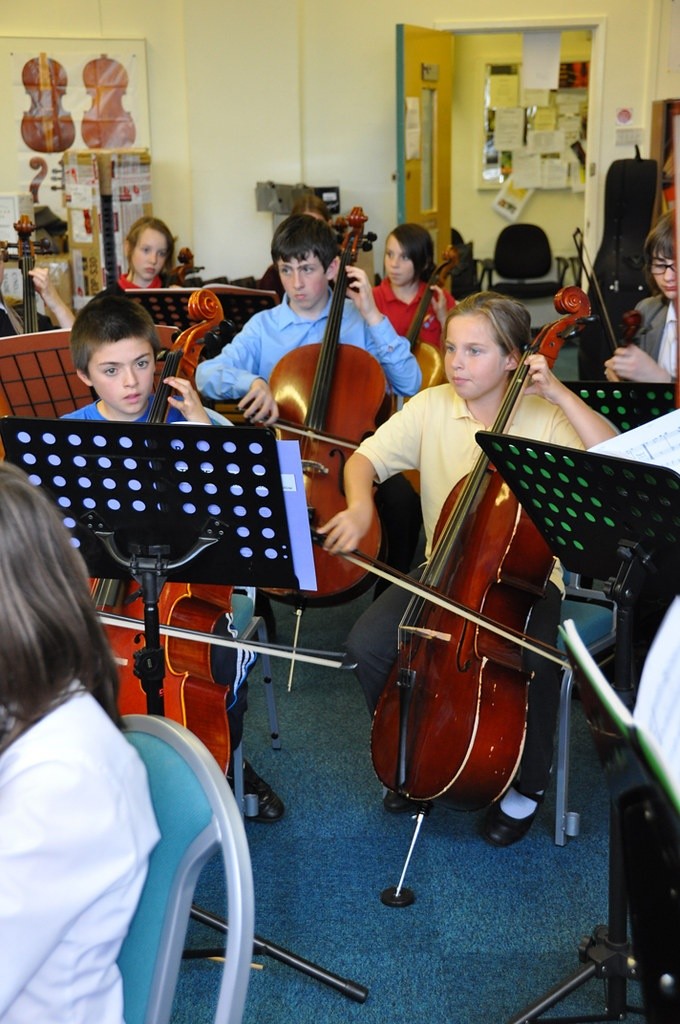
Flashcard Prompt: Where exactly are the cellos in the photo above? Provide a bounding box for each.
[253,207,386,693]
[11,216,41,334]
[372,282,592,906]
[169,249,196,287]
[382,244,463,498]
[85,292,234,783]
[616,311,644,349]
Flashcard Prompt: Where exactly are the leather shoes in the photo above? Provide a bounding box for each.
[485,781,548,845]
[383,792,412,814]
[225,760,284,821]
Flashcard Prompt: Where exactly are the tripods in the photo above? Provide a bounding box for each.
[473,430,679,1024]
[0,415,367,1000]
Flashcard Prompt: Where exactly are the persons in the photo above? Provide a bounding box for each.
[0,257,76,337]
[292,195,334,226]
[317,290,621,848]
[59,296,285,823]
[118,215,184,289]
[370,224,456,350]
[0,461,163,1024]
[195,214,422,639]
[605,210,677,384]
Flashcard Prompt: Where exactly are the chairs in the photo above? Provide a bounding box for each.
[114,222,617,1024]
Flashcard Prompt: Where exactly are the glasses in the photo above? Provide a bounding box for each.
[649,263,676,275]
